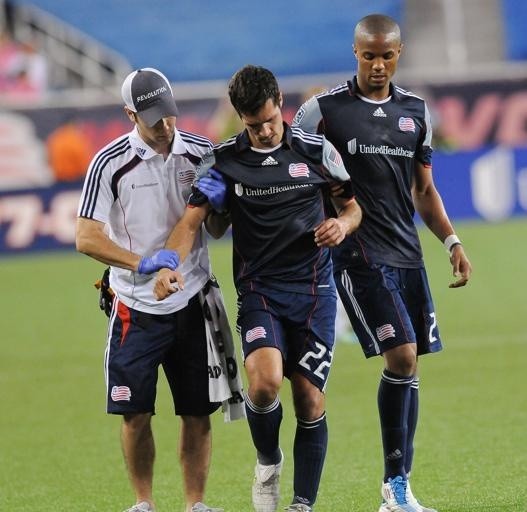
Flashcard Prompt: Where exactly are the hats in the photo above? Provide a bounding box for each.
[121,67,179,129]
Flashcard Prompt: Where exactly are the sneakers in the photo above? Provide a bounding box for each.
[125,502,154,512]
[192,502,224,512]
[287,503,312,512]
[379,471,436,512]
[252,448,283,512]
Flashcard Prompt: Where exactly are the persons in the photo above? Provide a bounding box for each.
[288,11,472,512]
[149,63,366,511]
[70,63,233,511]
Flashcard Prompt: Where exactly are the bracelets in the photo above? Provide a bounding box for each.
[441,234,462,252]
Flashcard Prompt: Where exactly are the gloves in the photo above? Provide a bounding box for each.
[138,248,179,275]
[198,168,227,210]
